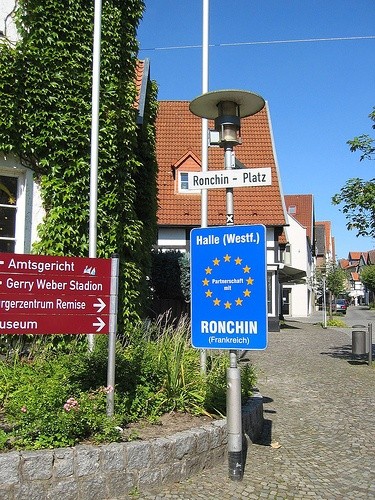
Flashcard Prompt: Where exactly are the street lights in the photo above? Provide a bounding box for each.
[186,87,267,480]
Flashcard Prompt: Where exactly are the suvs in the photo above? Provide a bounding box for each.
[330,299,346,315]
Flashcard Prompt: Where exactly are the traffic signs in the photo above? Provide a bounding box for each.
[0,253,111,333]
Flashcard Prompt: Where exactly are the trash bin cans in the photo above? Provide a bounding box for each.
[351,325,366,328]
[351,331,367,355]
